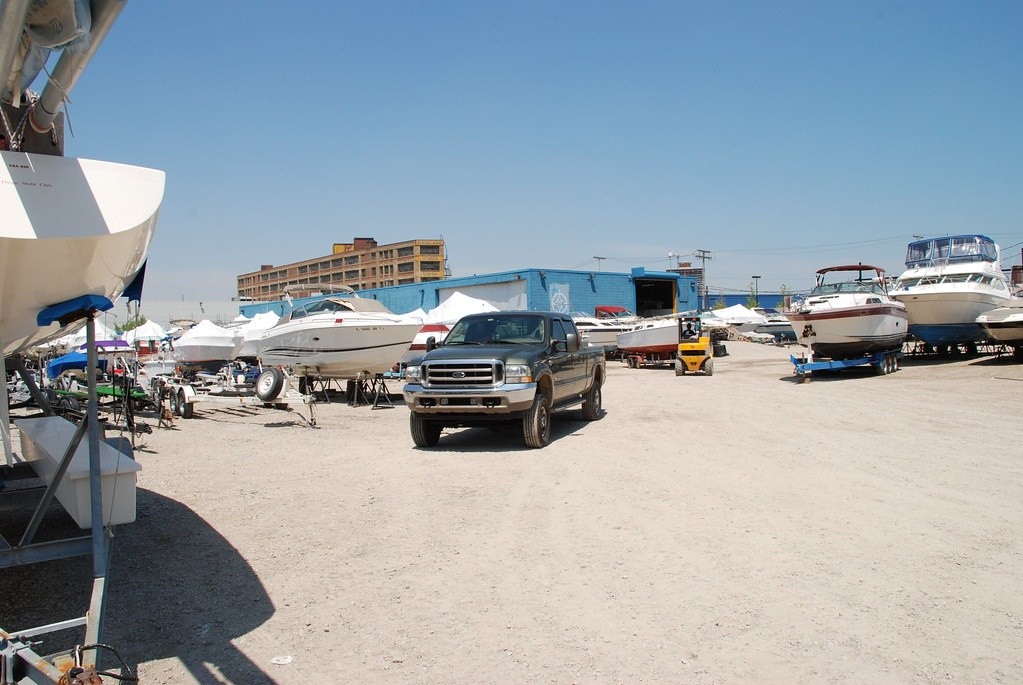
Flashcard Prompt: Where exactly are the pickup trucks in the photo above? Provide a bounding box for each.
[402,308,605,449]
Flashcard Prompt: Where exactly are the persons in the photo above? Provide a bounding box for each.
[158,380,176,429]
[681,323,696,339]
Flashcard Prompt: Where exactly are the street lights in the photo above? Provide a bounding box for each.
[752,275,763,307]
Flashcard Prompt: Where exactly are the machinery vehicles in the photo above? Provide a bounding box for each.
[675,316,714,376]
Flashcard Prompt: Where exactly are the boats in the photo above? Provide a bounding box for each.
[782,262,910,355]
[0,0,169,351]
[574,309,679,368]
[707,303,798,342]
[888,234,1013,346]
[974,283,1023,347]
[43,289,501,381]
